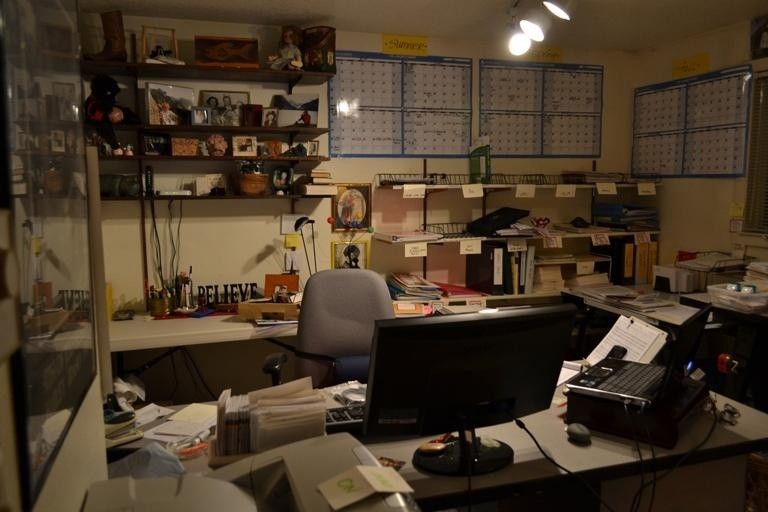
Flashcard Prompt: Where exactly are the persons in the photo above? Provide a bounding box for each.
[264,111,276,127]
[206,95,244,119]
[267,29,304,71]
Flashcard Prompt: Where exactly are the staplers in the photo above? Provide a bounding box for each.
[105,411,144,448]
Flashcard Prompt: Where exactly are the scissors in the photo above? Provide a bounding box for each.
[537,217,550,228]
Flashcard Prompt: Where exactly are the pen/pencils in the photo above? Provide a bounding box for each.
[180,265,192,307]
[192,425,217,446]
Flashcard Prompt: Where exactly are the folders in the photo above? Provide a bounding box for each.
[464,244,503,296]
[374,231,443,243]
[592,202,657,218]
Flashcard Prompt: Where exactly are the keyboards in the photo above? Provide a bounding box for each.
[326,405,365,429]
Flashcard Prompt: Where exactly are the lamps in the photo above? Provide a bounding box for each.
[294,216,318,275]
[505,0,585,56]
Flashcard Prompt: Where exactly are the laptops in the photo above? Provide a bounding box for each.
[566,303,712,409]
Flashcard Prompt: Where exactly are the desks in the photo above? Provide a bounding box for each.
[53,299,580,356]
[559,251,768,406]
[29,390,768,510]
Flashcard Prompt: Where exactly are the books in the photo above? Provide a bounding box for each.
[743,262,768,282]
[387,272,443,301]
[216,389,250,455]
[576,284,675,313]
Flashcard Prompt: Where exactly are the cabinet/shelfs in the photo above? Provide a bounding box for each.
[12,49,86,204]
[84,50,336,199]
[373,172,662,298]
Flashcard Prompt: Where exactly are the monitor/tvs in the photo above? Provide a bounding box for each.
[365,304,576,477]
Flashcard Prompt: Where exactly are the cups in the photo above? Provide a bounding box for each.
[166,435,209,474]
[148,299,171,317]
[177,287,193,308]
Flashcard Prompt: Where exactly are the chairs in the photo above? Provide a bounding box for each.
[261,267,396,389]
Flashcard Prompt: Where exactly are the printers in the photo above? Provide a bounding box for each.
[81,431,421,511]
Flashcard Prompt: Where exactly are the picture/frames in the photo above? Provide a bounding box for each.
[330,183,371,232]
[136,23,323,159]
[331,241,369,269]
